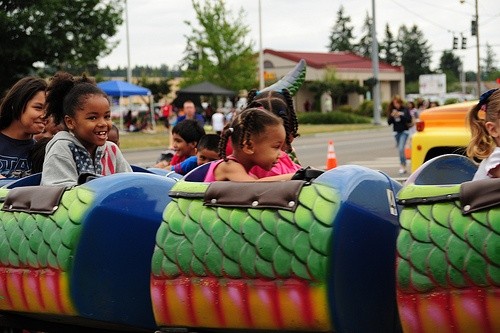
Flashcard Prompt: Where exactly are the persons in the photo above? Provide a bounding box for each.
[0,71,500,187]
[162,134,220,177]
[247,88,301,166]
[0,75,50,179]
[126,110,132,131]
[205,107,305,181]
[171,120,205,164]
[162,105,170,128]
[34,114,65,142]
[211,108,225,134]
[107,124,120,148]
[39,71,135,187]
[388,95,414,174]
[184,97,196,120]
[465,87,500,179]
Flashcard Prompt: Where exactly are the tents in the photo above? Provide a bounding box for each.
[97,80,156,132]
[175,81,237,110]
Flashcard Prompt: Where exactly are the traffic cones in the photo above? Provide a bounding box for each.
[405,134,412,159]
[324,139,338,170]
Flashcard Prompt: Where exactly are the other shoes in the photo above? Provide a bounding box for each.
[400,166,407,173]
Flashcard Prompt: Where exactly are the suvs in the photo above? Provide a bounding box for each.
[406,98,500,176]
